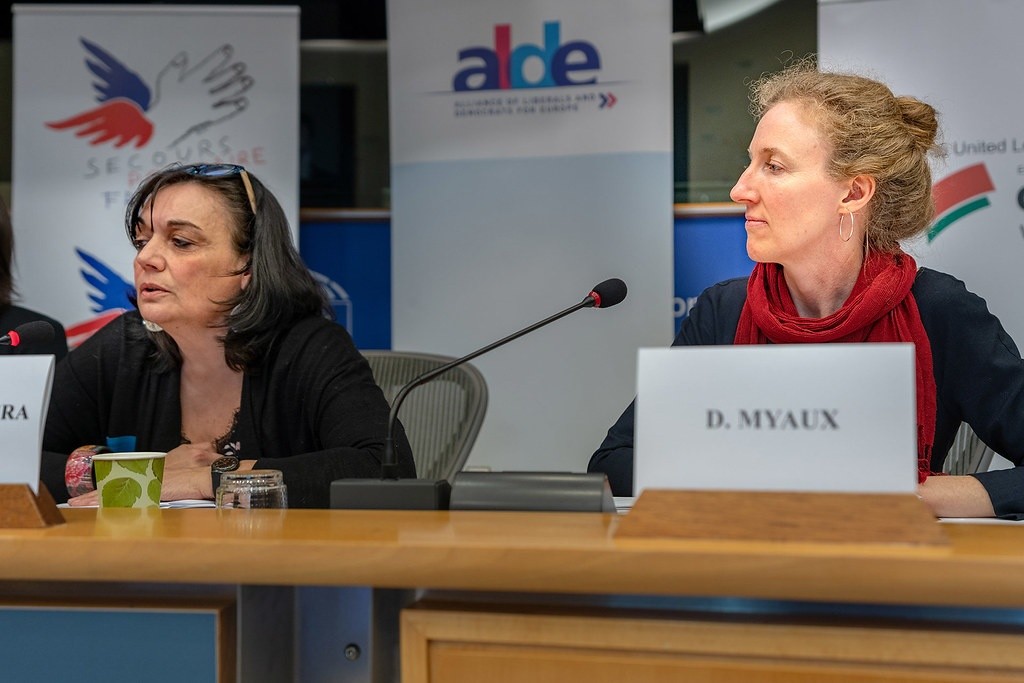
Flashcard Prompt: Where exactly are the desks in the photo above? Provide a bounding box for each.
[0,482,1024,683]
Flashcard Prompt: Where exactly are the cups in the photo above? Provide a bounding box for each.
[92,452,166,508]
[215,470,288,509]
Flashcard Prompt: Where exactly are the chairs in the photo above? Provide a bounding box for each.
[358,350,489,486]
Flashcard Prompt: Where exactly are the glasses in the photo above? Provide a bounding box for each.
[182,164,259,214]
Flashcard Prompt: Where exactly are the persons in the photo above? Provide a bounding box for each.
[0,186,69,365]
[39,162,417,510]
[588,69,1024,523]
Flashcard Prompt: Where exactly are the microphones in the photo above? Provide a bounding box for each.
[0,321,55,355]
[328,278,629,511]
